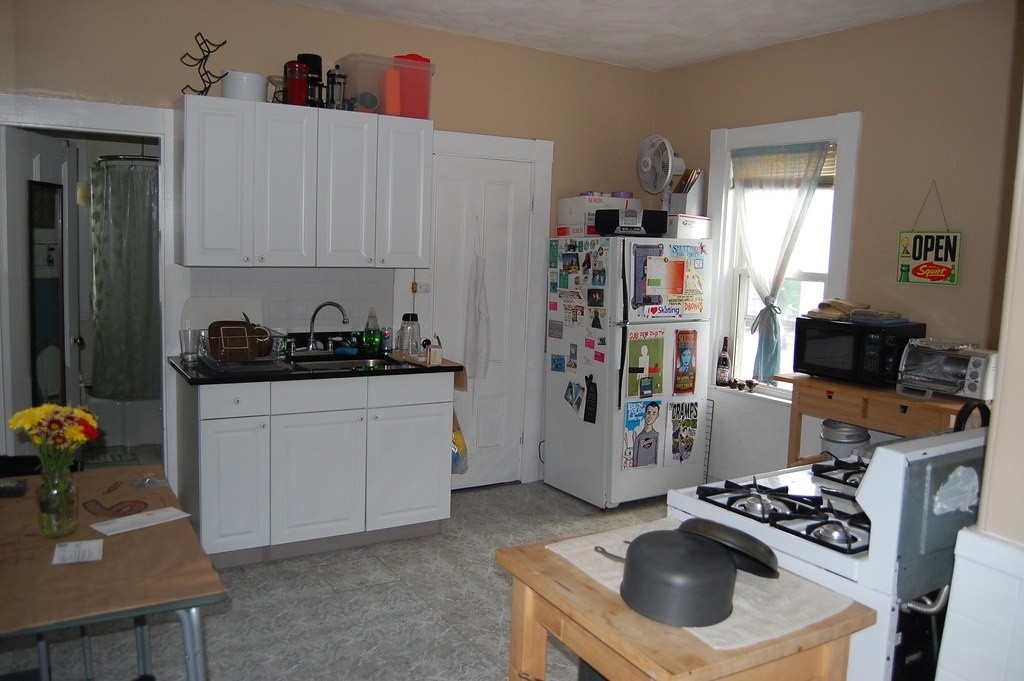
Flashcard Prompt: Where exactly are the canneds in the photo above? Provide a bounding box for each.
[380,327,393,351]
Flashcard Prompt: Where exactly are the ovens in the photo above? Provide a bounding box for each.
[897,338,998,401]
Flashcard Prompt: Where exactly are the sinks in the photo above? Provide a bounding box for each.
[295,358,392,371]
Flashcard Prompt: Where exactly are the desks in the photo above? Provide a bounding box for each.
[773,372,990,467]
[0,464,231,681]
[496,516,876,681]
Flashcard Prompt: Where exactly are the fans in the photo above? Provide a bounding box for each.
[635,133,686,211]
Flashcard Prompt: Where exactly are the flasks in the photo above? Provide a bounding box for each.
[284,61,309,107]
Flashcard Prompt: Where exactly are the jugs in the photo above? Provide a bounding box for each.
[298,53,348,109]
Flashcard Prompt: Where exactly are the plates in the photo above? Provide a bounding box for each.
[208,320,257,361]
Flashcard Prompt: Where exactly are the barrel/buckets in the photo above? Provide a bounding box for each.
[221,67,268,102]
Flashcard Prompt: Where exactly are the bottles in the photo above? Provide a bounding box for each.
[716,337,731,386]
[351,306,380,353]
[402,314,421,354]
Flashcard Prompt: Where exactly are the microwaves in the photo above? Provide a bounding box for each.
[794,314,926,390]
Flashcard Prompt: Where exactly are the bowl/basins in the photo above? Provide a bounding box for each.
[254,326,272,357]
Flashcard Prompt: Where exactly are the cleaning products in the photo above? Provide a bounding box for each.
[362,306,381,351]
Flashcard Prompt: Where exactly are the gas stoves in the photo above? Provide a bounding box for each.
[666,421,993,603]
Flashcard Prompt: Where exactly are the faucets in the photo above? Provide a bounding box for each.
[306,301,350,350]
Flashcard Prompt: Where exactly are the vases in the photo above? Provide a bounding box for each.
[35,472,78,538]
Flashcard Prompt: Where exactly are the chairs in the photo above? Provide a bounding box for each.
[0,456,94,681]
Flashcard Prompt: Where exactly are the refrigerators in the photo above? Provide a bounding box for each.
[542,236,716,511]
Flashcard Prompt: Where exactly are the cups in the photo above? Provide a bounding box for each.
[179,328,201,368]
[395,332,410,353]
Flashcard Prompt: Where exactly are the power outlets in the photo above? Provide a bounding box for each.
[411,281,431,293]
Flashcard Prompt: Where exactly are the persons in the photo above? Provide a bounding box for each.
[591,292,602,307]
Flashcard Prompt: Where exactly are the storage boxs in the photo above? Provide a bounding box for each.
[557,196,643,235]
[337,52,436,119]
[668,215,712,240]
[670,168,706,214]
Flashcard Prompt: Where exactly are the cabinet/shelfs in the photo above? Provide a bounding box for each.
[174,94,434,269]
[176,371,455,556]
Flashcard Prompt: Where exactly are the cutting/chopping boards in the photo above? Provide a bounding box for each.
[182,296,261,329]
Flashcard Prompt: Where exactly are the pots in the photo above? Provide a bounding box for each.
[819,420,871,459]
[594,518,778,627]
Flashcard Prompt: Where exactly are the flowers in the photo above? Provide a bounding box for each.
[9,403,99,469]
